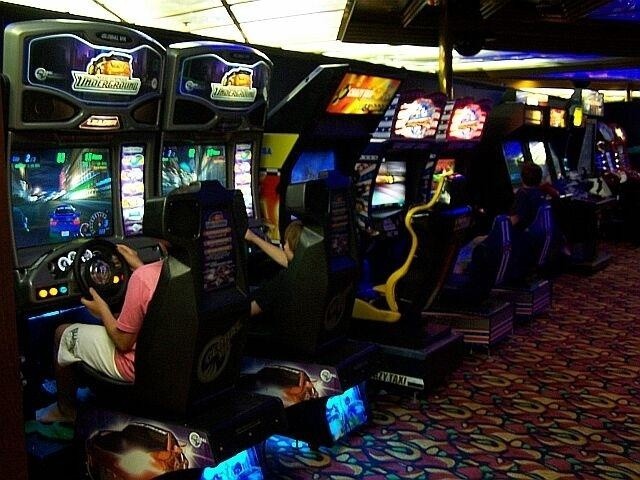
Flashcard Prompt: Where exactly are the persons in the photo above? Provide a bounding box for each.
[33,237,191,425]
[241,219,302,321]
[506,162,545,234]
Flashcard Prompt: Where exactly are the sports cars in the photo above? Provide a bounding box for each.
[13,207,34,235]
[47,203,83,239]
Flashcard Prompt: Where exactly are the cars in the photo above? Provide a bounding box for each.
[240,360,318,409]
[83,423,189,480]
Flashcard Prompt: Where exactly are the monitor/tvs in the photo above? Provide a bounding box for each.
[431,158,456,191]
[503,140,526,187]
[290,149,336,186]
[371,160,408,205]
[529,141,554,186]
[161,143,227,196]
[11,147,113,250]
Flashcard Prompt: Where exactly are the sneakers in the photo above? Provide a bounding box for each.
[35,408,77,424]
